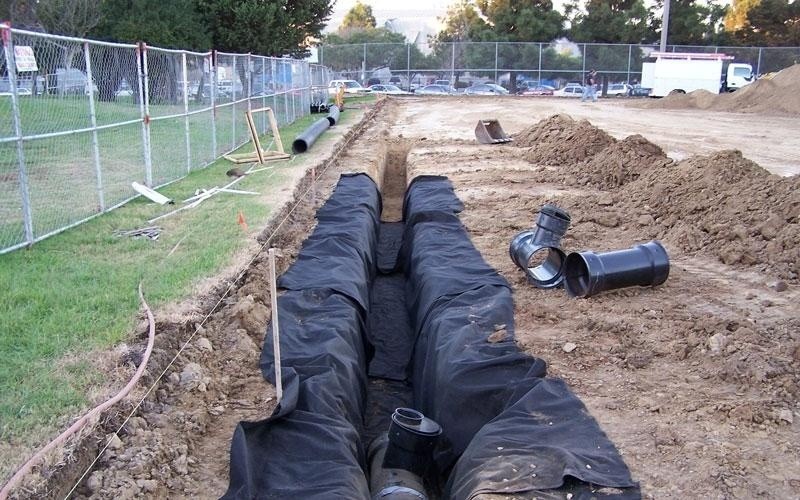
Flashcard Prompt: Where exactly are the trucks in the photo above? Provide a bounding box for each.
[640,51,757,99]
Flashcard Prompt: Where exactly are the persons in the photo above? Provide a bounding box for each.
[626,85,633,96]
[580,68,598,102]
